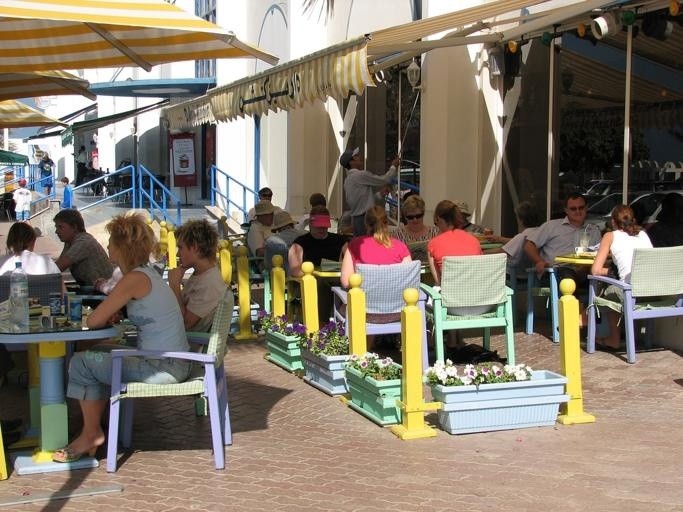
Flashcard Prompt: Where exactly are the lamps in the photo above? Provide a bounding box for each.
[407,57,423,92]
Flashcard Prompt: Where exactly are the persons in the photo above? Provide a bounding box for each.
[295,193,327,232]
[13,178,31,222]
[76,145,86,187]
[51,213,194,464]
[270,211,308,247]
[60,177,73,210]
[247,201,278,274]
[38,152,55,208]
[484,202,544,289]
[68,218,225,433]
[288,207,353,329]
[94,208,149,296]
[72,149,81,160]
[585,205,661,351]
[339,206,412,352]
[0,222,67,299]
[248,188,281,223]
[86,161,93,169]
[396,195,440,243]
[340,147,400,236]
[524,192,602,338]
[53,210,113,292]
[427,200,493,352]
[454,201,484,234]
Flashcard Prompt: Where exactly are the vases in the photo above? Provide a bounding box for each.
[300,349,349,395]
[264,323,301,373]
[345,361,403,427]
[431,370,570,434]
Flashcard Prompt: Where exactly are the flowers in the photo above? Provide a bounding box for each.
[259,310,309,338]
[345,352,403,381]
[294,317,349,356]
[422,358,534,387]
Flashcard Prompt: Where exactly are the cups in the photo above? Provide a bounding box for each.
[580,232,589,247]
[575,247,585,253]
[49,293,61,316]
[484,226,494,243]
[64,292,81,322]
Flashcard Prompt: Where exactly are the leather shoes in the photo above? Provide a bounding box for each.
[2,431,21,445]
[1,418,21,431]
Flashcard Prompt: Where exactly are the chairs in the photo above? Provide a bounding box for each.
[107,291,234,472]
[506,243,529,328]
[264,270,288,313]
[186,331,211,416]
[586,246,683,364]
[525,236,560,343]
[0,274,62,306]
[329,261,429,374]
[249,256,265,279]
[420,253,515,366]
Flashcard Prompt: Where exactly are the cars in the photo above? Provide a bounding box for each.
[385,158,421,208]
[580,179,615,195]
[583,190,682,245]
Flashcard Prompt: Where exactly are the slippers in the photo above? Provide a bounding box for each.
[52,443,98,462]
[596,338,612,351]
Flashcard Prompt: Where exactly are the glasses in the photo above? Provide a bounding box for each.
[260,193,272,197]
[453,200,471,216]
[566,205,586,210]
[406,213,424,220]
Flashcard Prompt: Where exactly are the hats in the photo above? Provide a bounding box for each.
[340,147,359,167]
[271,211,294,231]
[309,215,331,228]
[18,179,26,185]
[254,201,275,215]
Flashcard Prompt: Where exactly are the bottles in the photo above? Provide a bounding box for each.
[9,262,29,327]
